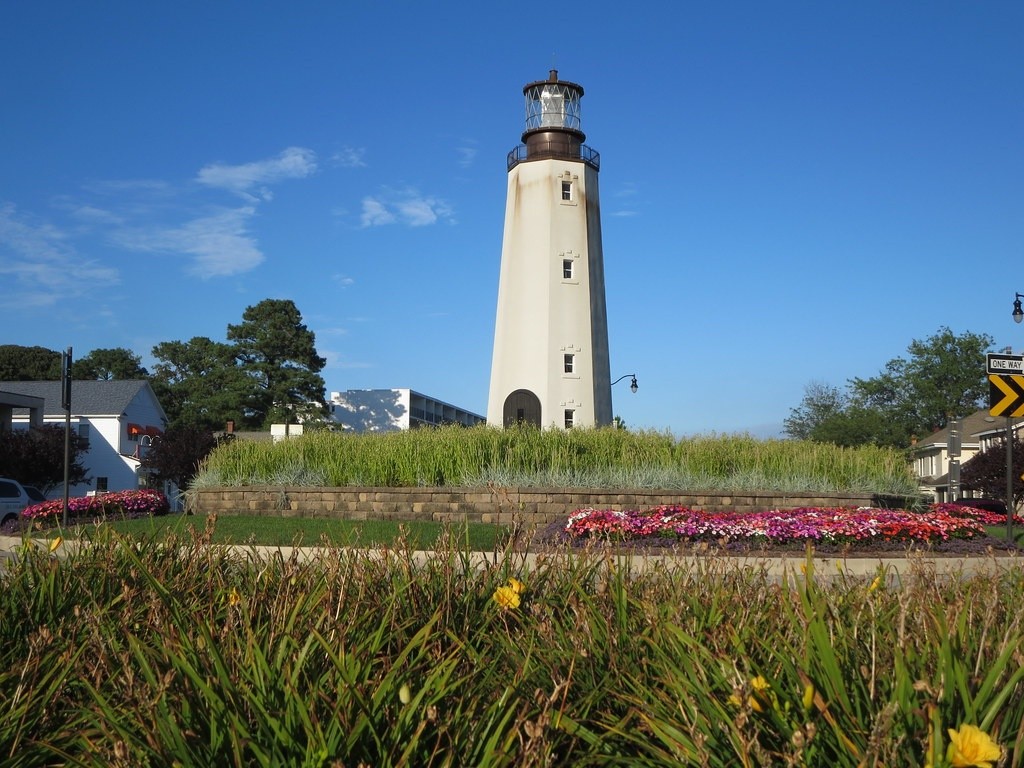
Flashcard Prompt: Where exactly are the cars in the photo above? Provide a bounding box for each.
[0,478,37,528]
